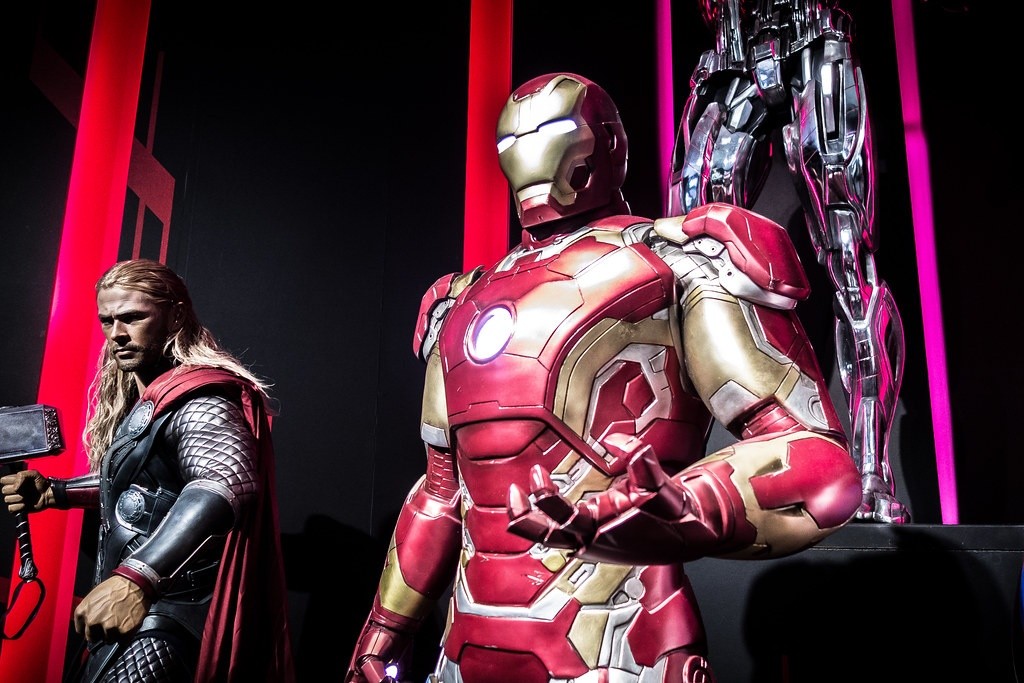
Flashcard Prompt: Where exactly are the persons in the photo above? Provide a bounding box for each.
[668,0,910,524]
[0,261,295,683]
[346,72,864,683]
